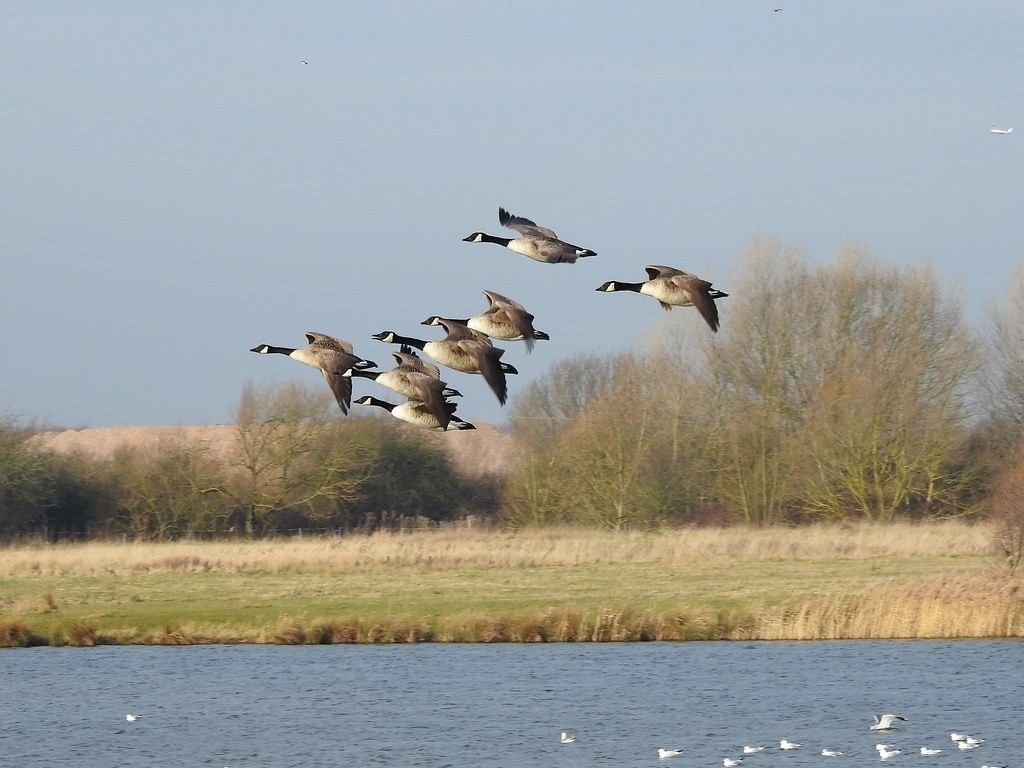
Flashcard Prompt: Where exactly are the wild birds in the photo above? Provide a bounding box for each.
[462,206,598,264]
[919,747,944,756]
[868,713,909,730]
[595,264,730,335]
[657,748,685,760]
[743,746,766,755]
[126,714,142,723]
[779,740,804,749]
[420,290,550,358]
[981,765,1010,768]
[821,749,846,758]
[950,732,987,750]
[352,396,477,432]
[332,343,464,430]
[876,744,904,758]
[560,732,576,744]
[249,331,378,416]
[372,319,518,408]
[721,758,745,768]
